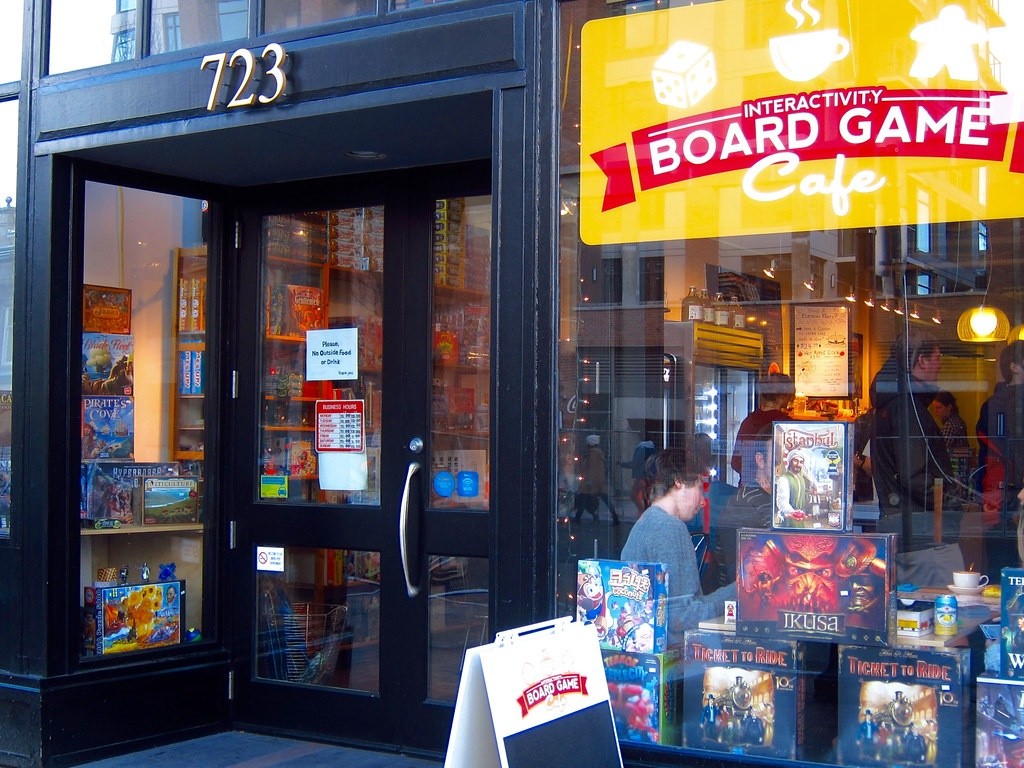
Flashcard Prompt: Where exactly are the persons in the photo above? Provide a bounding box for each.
[559,435,618,526]
[622,447,736,651]
[631,430,657,519]
[714,423,776,587]
[852,330,970,587]
[731,373,799,475]
[976,340,1024,568]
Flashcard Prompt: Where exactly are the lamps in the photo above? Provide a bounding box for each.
[803,273,817,291]
[910,304,920,319]
[880,296,892,312]
[932,309,942,324]
[893,300,905,314]
[958,223,1024,347]
[864,292,875,307]
[763,259,778,279]
[845,286,856,302]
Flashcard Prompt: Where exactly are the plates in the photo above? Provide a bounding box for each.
[946,585,985,594]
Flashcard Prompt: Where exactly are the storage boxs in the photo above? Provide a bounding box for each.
[171,198,489,644]
[81,280,206,529]
[575,422,1023,768]
[84,579,186,655]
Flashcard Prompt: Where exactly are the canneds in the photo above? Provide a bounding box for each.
[933,594,959,636]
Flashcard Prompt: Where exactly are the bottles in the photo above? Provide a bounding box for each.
[712,293,729,326]
[681,286,704,324]
[699,289,715,325]
[694,290,700,299]
[728,297,746,331]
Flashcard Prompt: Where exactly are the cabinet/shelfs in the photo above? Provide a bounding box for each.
[173,243,490,652]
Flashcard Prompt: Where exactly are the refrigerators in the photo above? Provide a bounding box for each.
[664,319,764,487]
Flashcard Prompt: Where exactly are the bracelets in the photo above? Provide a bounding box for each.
[856,451,866,470]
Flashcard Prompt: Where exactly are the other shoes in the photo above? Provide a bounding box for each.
[609,506,620,527]
[571,509,584,523]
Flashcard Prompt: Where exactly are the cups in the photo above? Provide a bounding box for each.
[952,571,989,588]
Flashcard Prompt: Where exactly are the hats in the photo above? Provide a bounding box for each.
[586,434,600,446]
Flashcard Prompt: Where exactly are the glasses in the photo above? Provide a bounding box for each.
[923,354,943,362]
[933,405,948,410]
